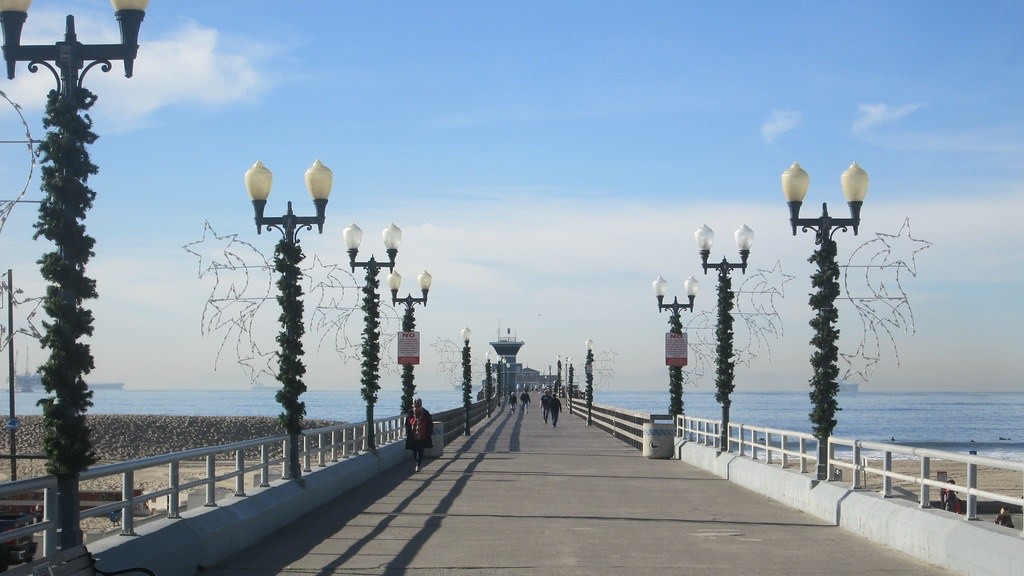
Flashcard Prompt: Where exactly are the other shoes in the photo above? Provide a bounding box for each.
[552,423,556,427]
[415,465,420,472]
[544,420,547,423]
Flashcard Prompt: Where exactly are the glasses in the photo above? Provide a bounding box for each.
[414,405,420,407]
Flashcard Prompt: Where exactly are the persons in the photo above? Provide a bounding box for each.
[995,507,1014,528]
[405,398,433,472]
[547,393,562,428]
[944,480,956,513]
[520,390,531,414]
[33,505,42,523]
[540,390,552,424]
[508,392,517,413]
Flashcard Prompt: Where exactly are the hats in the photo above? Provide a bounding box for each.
[1001,507,1010,516]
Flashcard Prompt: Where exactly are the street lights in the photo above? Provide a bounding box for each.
[294,221,402,451]
[2,1,149,558]
[316,269,432,435]
[180,158,334,481]
[694,220,797,452]
[471,348,585,415]
[584,338,619,426]
[780,157,936,481]
[429,326,474,436]
[651,273,719,435]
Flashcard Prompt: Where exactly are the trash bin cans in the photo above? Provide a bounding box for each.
[422,422,444,458]
[642,423,675,459]
